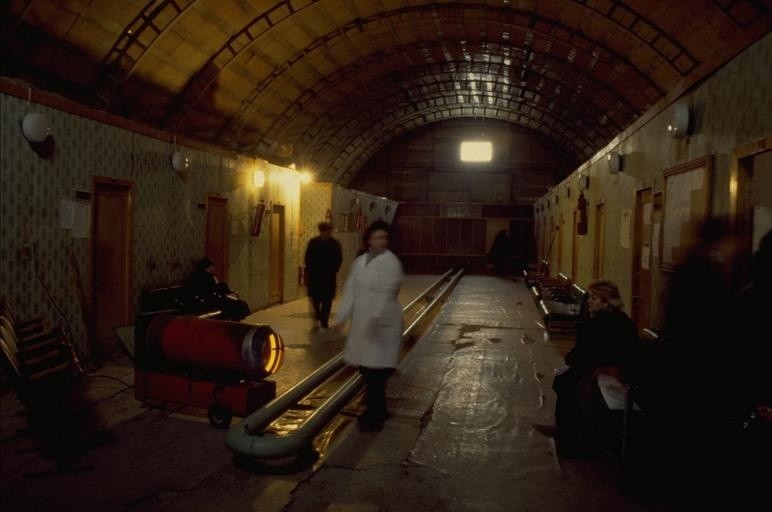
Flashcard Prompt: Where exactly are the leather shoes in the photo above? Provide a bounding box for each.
[534,422,557,438]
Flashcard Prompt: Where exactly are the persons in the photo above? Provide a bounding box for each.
[185,256,253,322]
[487,225,512,277]
[623,213,772,512]
[302,221,344,329]
[531,275,637,440]
[332,219,405,433]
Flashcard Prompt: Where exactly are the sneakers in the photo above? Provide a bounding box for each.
[357,408,388,429]
[314,313,328,328]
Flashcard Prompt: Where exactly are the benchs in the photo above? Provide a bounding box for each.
[141,284,241,324]
[522,270,589,333]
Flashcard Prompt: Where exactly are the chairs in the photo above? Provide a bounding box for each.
[1,303,81,433]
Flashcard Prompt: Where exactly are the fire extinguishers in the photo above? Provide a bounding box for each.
[251,198,265,237]
[576,194,587,235]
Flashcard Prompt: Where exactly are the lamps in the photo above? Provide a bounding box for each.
[172,151,189,172]
[666,101,690,137]
[607,151,620,175]
[24,112,49,141]
[254,170,266,187]
[578,174,587,190]
